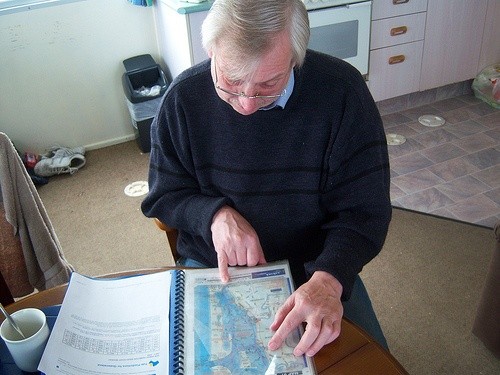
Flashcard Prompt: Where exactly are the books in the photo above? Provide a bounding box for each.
[37,260,318,375]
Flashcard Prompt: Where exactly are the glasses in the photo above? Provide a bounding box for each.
[215,50,292,100]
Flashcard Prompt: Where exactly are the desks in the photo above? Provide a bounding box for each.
[0,268,407,375]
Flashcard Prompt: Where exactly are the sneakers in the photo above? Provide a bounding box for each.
[40,146,85,159]
[34,154,86,177]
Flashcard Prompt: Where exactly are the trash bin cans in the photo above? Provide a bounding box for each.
[121,54,170,153]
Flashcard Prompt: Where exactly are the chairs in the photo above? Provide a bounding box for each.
[0,132,75,307]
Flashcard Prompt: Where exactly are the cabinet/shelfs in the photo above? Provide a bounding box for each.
[368,0,500,117]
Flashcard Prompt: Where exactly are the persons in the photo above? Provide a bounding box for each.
[141,0,392,357]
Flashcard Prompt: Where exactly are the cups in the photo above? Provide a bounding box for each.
[0,308,51,372]
